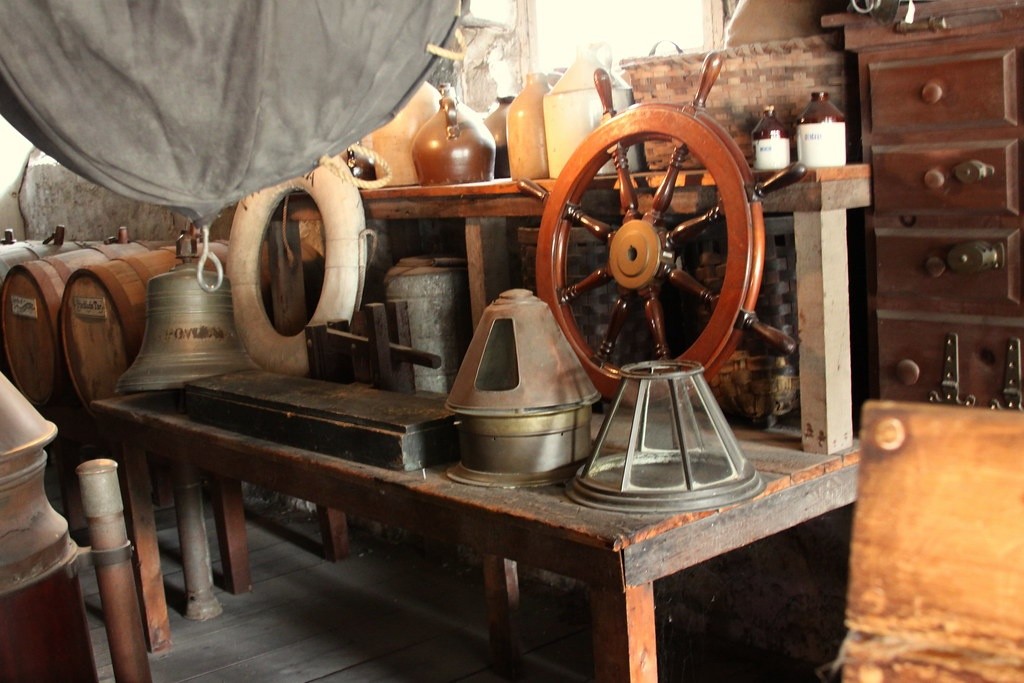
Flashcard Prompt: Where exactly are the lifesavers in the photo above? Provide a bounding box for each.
[225,155,369,378]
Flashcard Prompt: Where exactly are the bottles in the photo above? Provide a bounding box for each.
[506,73,560,182]
[754,103,789,167]
[484,96,515,179]
[797,91,846,167]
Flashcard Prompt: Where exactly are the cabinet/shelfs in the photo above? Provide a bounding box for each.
[821,0,1024,411]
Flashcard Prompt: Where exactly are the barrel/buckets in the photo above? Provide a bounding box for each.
[58,224,270,409]
[0,227,168,408]
[0,225,107,286]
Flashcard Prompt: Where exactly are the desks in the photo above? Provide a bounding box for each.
[265,166,872,455]
[90,391,860,683]
